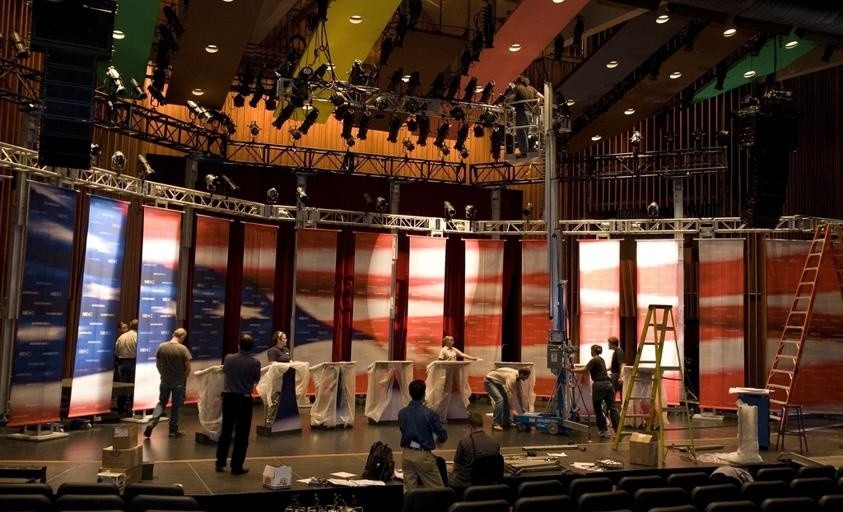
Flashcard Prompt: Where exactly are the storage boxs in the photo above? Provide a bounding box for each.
[262,464,292,490]
[102,446,143,467]
[630,432,659,466]
[112,425,138,448]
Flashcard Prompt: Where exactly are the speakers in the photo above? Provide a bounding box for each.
[31,0,116,170]
[738,115,798,227]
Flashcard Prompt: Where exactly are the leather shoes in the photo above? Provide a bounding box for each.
[231,466,250,476]
[215,462,225,474]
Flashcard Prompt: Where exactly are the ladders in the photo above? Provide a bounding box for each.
[612,306,696,466]
[765,225,843,433]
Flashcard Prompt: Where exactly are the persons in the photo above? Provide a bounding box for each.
[215,334,261,474]
[483,367,531,431]
[144,328,193,437]
[112,320,128,404]
[453,413,500,487]
[267,331,292,361]
[398,380,448,495]
[115,319,138,417]
[438,336,479,360]
[566,345,620,437]
[604,337,624,418]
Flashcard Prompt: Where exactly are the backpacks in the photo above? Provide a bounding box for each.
[364,441,395,485]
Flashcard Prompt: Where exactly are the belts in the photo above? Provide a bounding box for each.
[406,447,429,453]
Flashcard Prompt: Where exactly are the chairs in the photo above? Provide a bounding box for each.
[0,465,198,512]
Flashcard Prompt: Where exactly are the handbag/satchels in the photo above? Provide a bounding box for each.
[469,452,505,486]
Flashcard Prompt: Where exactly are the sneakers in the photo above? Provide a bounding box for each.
[491,424,502,431]
[596,430,611,439]
[169,428,186,437]
[142,424,152,439]
[506,421,516,428]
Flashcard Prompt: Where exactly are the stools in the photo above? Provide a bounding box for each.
[775,404,808,454]
